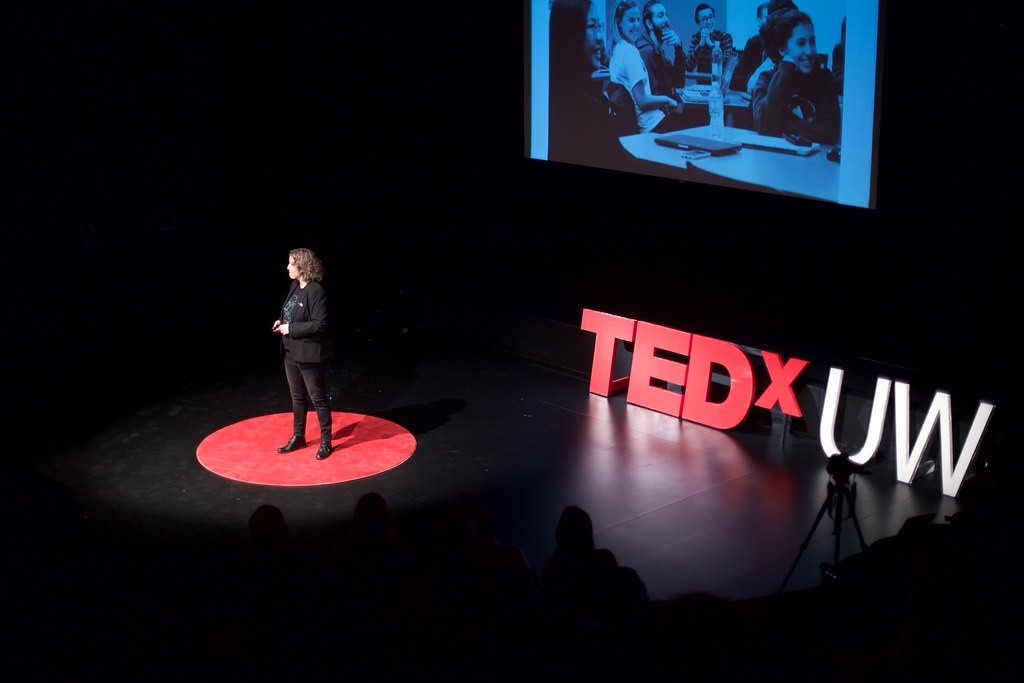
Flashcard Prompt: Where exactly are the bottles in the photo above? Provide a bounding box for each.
[709,82,724,141]
[712,41,722,75]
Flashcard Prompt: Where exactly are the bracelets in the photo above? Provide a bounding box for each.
[710,42,716,50]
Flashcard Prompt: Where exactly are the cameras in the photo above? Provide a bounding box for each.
[827,454,873,479]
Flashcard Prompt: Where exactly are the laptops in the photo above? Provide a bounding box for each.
[737,135,820,156]
[683,52,739,96]
[654,134,742,156]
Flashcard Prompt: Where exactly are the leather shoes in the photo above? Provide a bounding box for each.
[315,441,333,459]
[277,436,307,454]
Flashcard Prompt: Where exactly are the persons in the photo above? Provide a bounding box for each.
[638,0,686,132]
[685,3,734,96]
[729,0,799,91]
[548,0,641,169]
[608,0,679,135]
[271,247,335,459]
[751,7,841,143]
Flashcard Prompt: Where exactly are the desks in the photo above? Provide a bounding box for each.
[674,87,751,112]
[660,126,839,204]
[685,72,712,81]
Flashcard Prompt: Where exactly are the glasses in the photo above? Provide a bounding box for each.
[586,22,605,35]
[699,14,714,22]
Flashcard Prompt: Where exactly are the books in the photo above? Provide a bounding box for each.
[677,85,730,102]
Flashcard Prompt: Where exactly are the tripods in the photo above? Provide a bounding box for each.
[776,476,866,595]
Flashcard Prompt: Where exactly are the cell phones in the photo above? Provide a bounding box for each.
[783,133,812,146]
[681,149,711,160]
